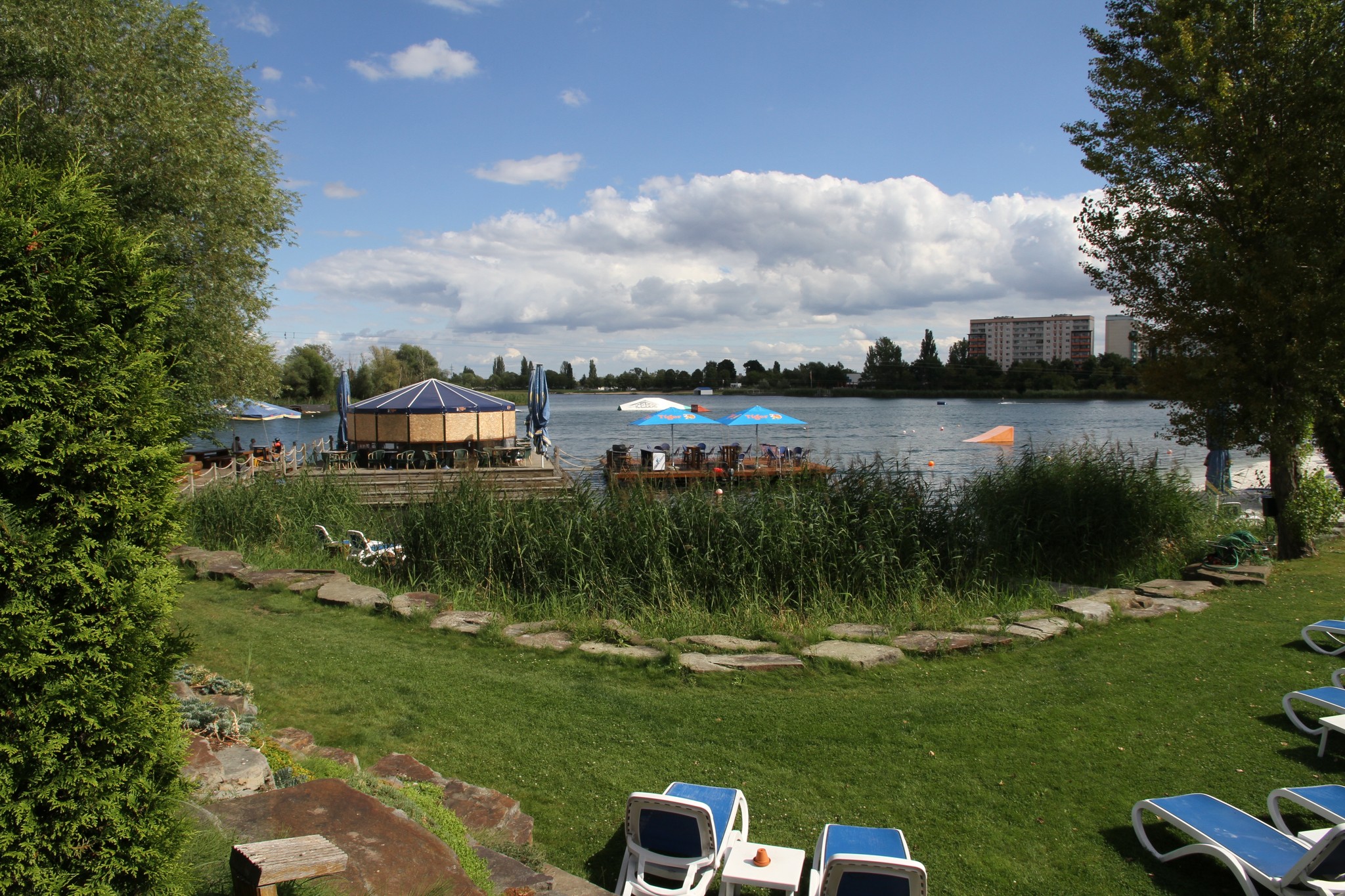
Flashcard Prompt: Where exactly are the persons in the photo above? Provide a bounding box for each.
[232,436,245,453]
[250,438,258,451]
[272,438,284,454]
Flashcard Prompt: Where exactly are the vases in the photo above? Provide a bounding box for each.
[755,848,769,863]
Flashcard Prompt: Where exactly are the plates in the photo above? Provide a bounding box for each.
[753,856,771,867]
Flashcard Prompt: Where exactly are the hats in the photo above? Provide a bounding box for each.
[275,438,280,441]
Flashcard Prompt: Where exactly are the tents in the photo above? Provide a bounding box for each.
[346,378,516,464]
[195,396,302,458]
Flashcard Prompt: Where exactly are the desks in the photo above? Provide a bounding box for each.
[722,445,737,469]
[233,448,275,466]
[518,442,532,446]
[382,451,398,470]
[515,438,524,441]
[1317,714,1345,758]
[686,446,701,468]
[640,449,666,471]
[722,841,805,896]
[438,450,454,469]
[186,448,227,466]
[498,447,525,465]
[617,456,633,474]
[327,451,348,469]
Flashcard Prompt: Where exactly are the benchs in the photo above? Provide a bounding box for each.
[204,454,279,468]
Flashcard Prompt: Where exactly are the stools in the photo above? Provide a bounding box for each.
[681,451,688,468]
[684,452,692,468]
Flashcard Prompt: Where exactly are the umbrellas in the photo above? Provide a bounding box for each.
[616,398,694,446]
[714,406,809,469]
[627,407,722,472]
[525,363,552,467]
[337,370,351,450]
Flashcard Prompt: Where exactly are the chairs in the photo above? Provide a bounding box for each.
[1131,784,1345,896]
[614,782,927,896]
[321,437,532,471]
[1281,619,1345,735]
[606,442,810,472]
[312,524,406,568]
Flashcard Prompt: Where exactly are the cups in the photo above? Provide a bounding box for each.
[755,848,770,864]
